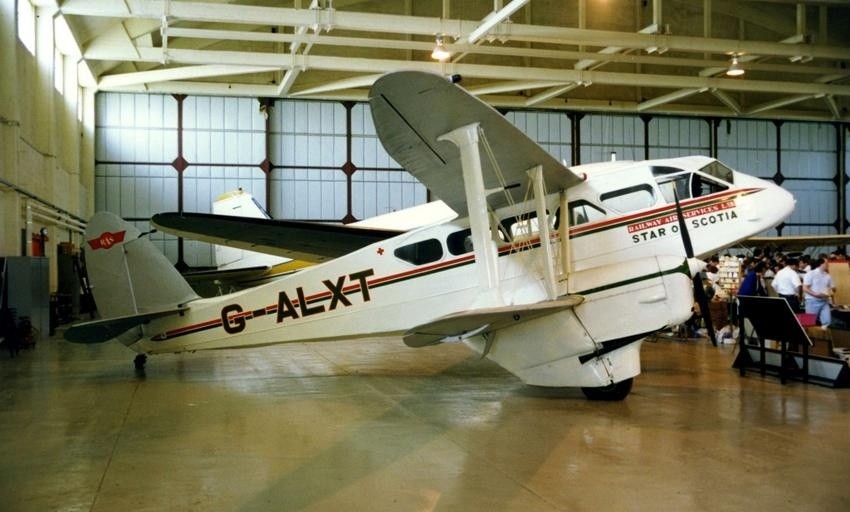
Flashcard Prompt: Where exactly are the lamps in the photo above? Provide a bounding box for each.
[430,32,453,60]
[725,50,746,78]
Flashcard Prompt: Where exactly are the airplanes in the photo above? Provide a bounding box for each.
[57,68,800,403]
[729,234,850,308]
[180,155,638,290]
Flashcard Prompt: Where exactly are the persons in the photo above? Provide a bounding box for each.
[679,248,850,337]
